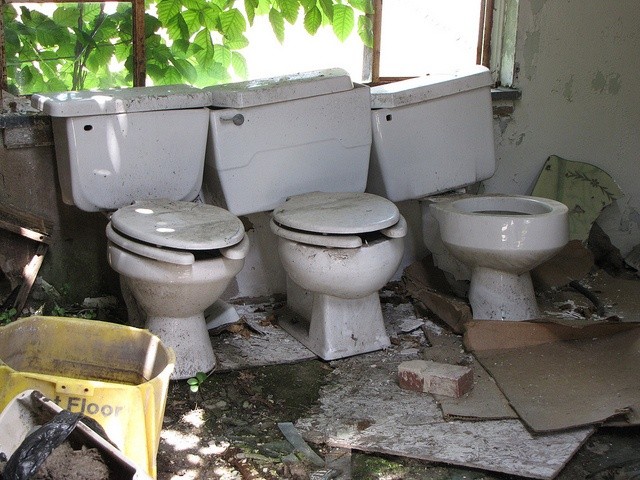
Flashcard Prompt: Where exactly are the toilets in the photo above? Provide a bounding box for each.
[369,64,571,322]
[30,85,250,382]
[202,67,408,360]
